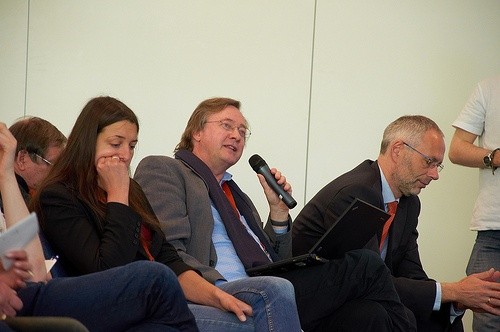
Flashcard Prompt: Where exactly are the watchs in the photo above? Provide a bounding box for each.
[482,147,499,176]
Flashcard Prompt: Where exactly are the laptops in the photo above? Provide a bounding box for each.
[247,197,393,275]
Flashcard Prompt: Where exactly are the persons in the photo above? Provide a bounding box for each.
[0,115,201,332]
[26,96,303,332]
[134,97,418,332]
[447,75,500,332]
[293,115,500,332]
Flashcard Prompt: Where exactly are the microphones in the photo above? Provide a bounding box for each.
[248,154,297,210]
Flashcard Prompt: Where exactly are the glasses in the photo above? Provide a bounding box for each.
[206,120,251,138]
[403,142,445,173]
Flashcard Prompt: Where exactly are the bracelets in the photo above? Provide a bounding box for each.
[271,220,289,226]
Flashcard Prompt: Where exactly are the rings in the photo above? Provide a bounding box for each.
[487,297,491,304]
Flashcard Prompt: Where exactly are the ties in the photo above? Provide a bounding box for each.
[379,201,398,255]
